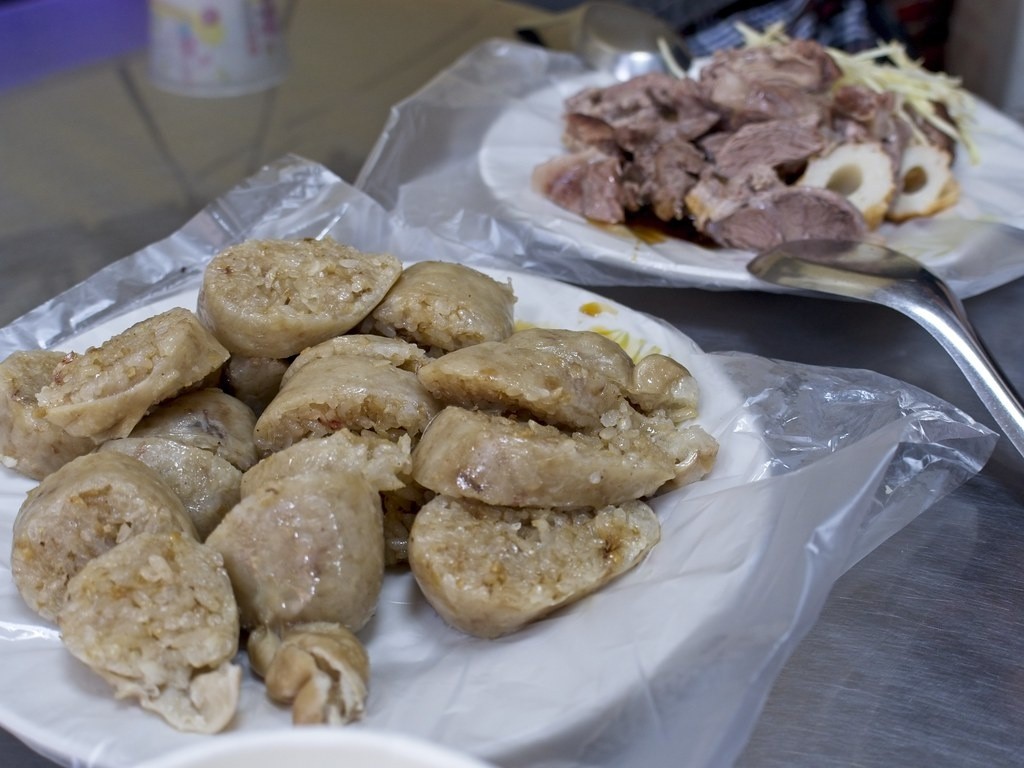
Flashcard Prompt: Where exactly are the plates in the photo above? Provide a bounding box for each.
[481,56,1024,300]
[0,262,774,768]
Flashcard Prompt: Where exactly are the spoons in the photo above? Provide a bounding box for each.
[574,0,690,84]
[746,239,1024,459]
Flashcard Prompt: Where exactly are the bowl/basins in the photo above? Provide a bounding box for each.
[137,726,496,768]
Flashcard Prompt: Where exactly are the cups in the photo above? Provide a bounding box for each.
[147,0,288,98]
